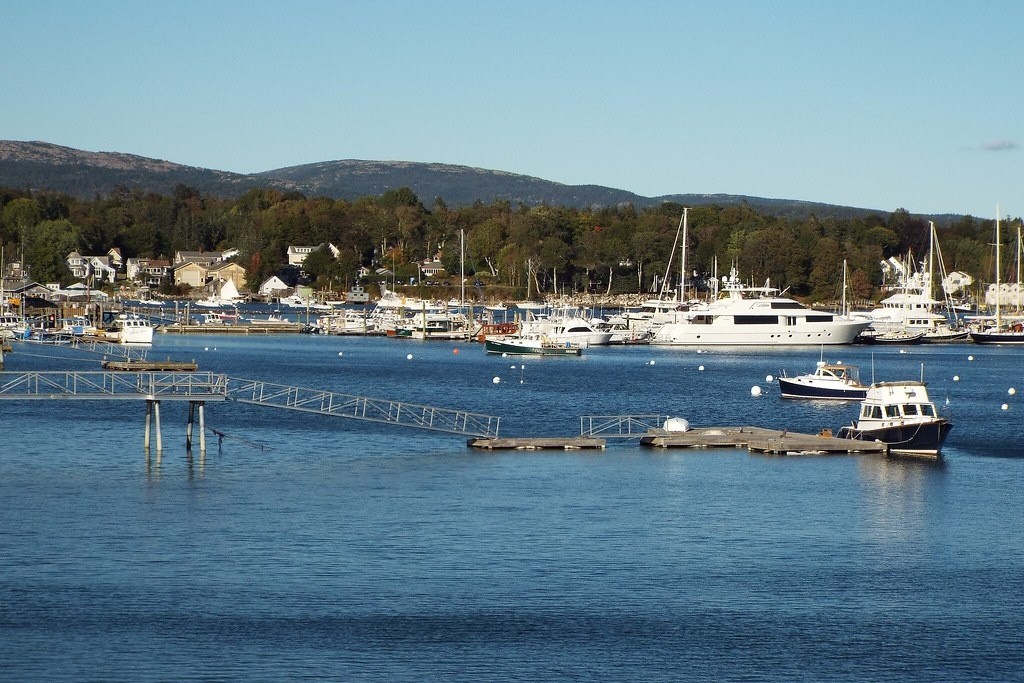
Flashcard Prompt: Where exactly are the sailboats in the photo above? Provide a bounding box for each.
[905,219,971,341]
[969,205,1024,343]
[646,207,698,332]
[828,260,883,337]
[442,230,489,333]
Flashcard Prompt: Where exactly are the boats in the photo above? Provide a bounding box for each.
[195,295,242,307]
[518,259,650,344]
[654,259,874,344]
[372,253,451,330]
[872,320,926,344]
[605,279,704,333]
[0,309,24,330]
[201,313,225,325]
[867,247,947,329]
[314,308,377,331]
[106,307,153,343]
[485,336,583,356]
[840,351,955,456]
[486,301,508,311]
[776,343,870,400]
[245,292,298,325]
